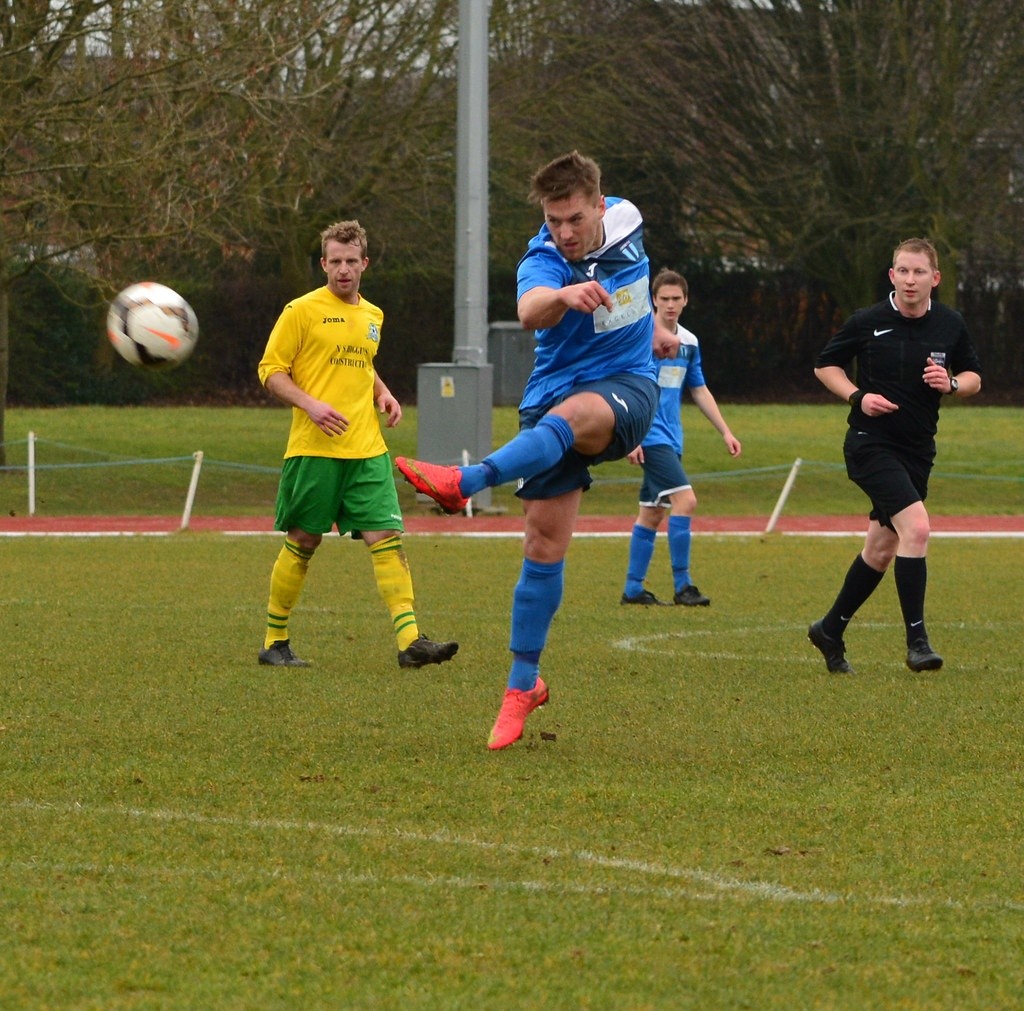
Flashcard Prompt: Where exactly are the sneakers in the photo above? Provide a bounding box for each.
[258,639,310,668]
[672,583,710,606]
[907,638,943,673]
[808,616,853,674]
[397,633,459,669]
[620,589,673,606]
[488,677,549,749]
[394,456,469,515]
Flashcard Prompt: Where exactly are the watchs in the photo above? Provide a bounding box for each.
[946,377,958,395]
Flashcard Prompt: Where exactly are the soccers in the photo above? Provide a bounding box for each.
[106,282,199,371]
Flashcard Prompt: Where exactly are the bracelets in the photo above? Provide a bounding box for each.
[849,390,867,411]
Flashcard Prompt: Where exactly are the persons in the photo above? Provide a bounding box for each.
[808,237,986,674]
[394,150,680,749]
[622,270,742,607]
[257,221,461,668]
